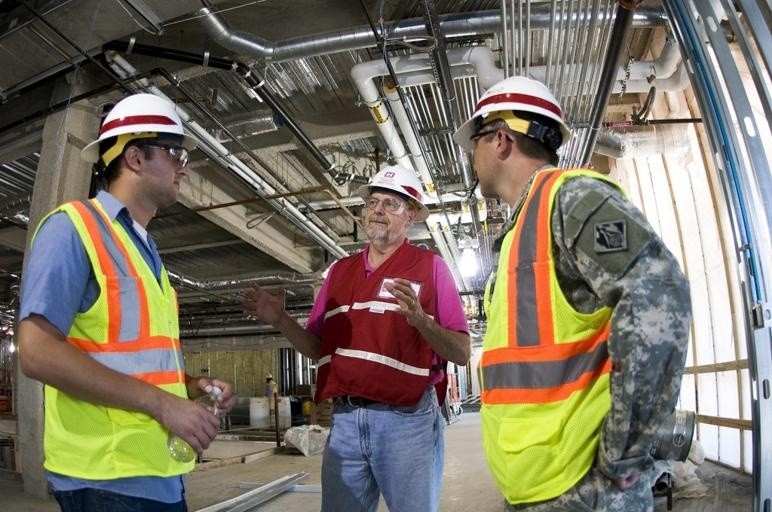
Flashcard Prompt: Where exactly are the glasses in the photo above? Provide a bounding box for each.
[468,128,515,143]
[364,195,415,217]
[138,137,193,168]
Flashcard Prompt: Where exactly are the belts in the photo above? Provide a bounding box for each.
[331,395,384,407]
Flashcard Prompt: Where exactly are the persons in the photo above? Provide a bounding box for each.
[264,373,280,396]
[17,93,239,512]
[454,75,694,512]
[241,163,473,512]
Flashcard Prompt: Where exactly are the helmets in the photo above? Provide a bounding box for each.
[451,75,572,154]
[264,373,273,380]
[76,91,199,167]
[356,163,431,223]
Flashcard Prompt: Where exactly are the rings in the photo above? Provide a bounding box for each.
[408,299,415,309]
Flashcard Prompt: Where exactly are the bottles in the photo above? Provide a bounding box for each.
[169,385,223,464]
